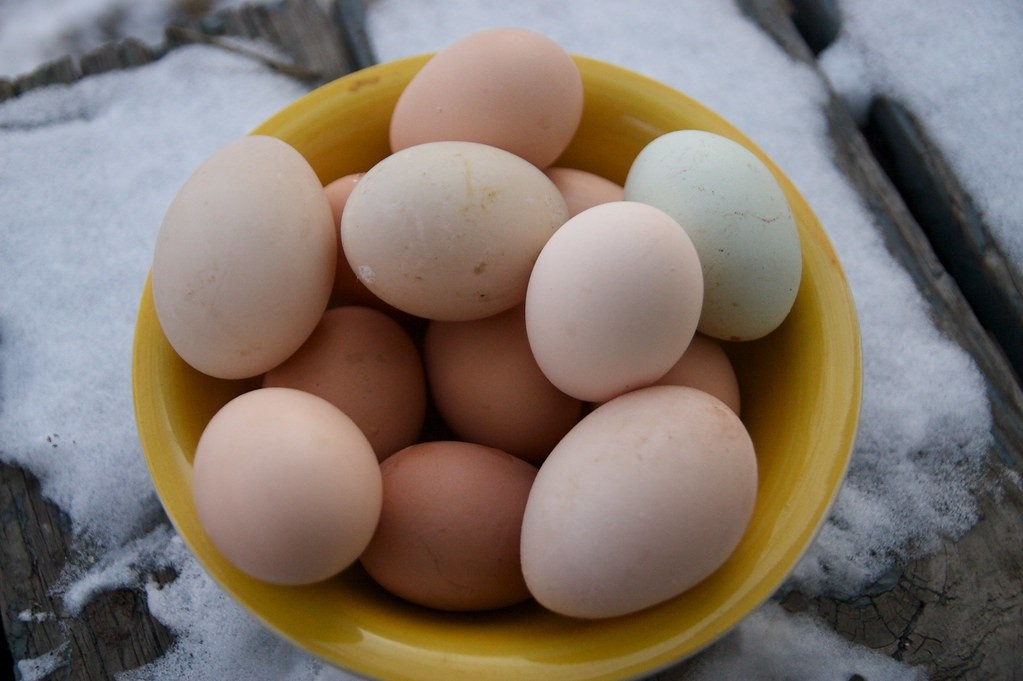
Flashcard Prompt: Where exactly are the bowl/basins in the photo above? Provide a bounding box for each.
[130,49,864,681]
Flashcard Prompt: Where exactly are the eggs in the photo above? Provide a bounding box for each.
[150,25,802,620]
